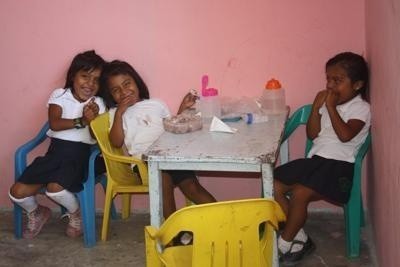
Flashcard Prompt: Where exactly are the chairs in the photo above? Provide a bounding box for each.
[281,103,373,257]
[14,108,119,248]
[144,197,287,267]
[89,109,193,240]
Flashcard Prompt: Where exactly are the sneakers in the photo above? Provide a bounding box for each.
[22,204,51,240]
[62,207,83,238]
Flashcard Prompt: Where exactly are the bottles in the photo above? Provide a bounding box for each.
[201,75,221,132]
[263,80,286,120]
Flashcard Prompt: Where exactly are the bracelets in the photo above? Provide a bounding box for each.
[73,117,85,129]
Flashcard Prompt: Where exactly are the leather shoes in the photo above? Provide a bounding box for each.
[274,235,291,260]
[288,234,316,262]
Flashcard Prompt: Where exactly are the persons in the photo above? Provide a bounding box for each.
[274,52,372,262]
[9,51,107,239]
[100,60,217,247]
